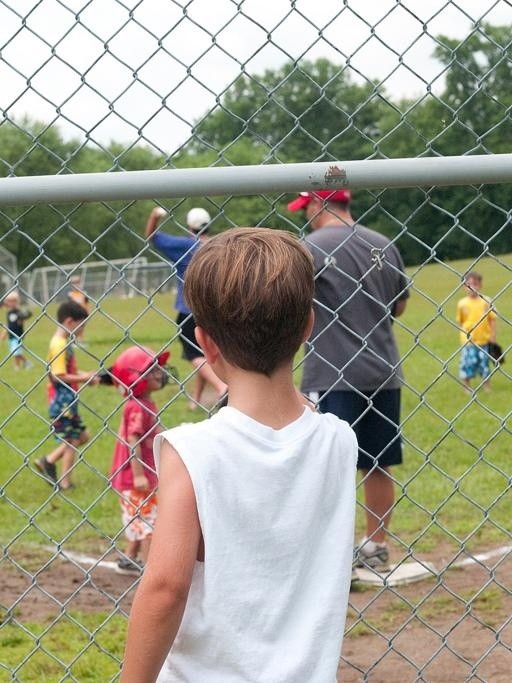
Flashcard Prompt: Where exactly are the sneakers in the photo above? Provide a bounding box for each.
[34,456,60,488]
[116,557,143,576]
[353,546,389,571]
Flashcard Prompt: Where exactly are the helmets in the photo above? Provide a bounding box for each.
[112,345,169,398]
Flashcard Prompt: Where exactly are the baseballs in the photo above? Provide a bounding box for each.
[158,206,167,216]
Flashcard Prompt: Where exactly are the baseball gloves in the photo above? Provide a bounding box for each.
[489,342,504,363]
[18,310,30,318]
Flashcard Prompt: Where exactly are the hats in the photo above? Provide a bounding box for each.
[186,207,211,231]
[286,188,352,210]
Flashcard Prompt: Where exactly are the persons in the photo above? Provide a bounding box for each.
[110,346,170,577]
[144,207,230,411]
[289,189,411,582]
[68,275,92,349]
[117,227,359,683]
[455,272,497,396]
[1,290,32,370]
[33,300,103,492]
